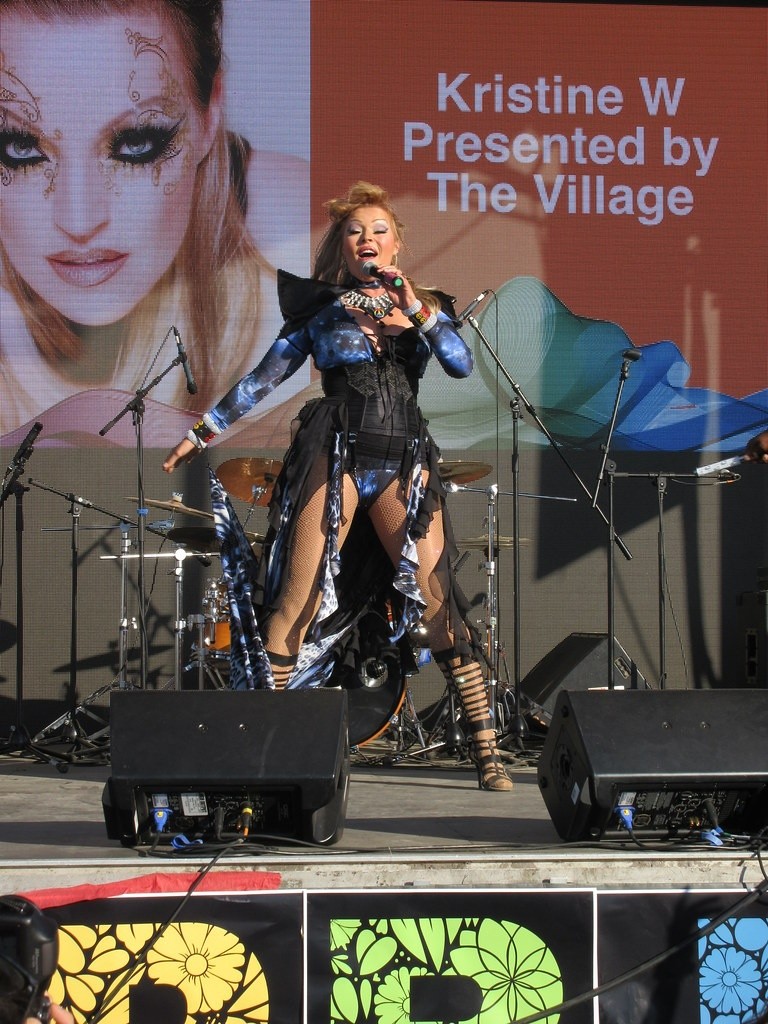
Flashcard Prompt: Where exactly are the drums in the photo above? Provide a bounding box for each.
[323,607,408,751]
[194,578,232,675]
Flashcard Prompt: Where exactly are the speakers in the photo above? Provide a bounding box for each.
[537,690,768,842]
[102,690,352,851]
[515,632,652,726]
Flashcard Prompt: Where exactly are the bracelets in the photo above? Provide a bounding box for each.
[184,413,221,452]
[401,299,438,334]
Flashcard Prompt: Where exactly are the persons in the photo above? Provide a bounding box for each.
[1,1,327,449]
[161,181,516,791]
[743,430,768,464]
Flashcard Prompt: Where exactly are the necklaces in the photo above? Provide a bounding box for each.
[347,276,383,289]
[340,290,396,320]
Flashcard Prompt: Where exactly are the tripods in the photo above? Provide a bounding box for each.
[0,446,186,774]
[380,312,634,770]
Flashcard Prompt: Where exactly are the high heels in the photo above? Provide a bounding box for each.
[469,736,514,793]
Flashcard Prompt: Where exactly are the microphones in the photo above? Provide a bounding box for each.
[174,327,198,396]
[456,290,489,322]
[720,469,742,481]
[191,548,212,568]
[361,260,404,289]
[2,424,43,475]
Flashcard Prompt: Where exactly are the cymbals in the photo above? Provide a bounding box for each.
[166,527,266,556]
[215,457,285,507]
[436,454,494,484]
[454,530,536,550]
[123,492,214,521]
